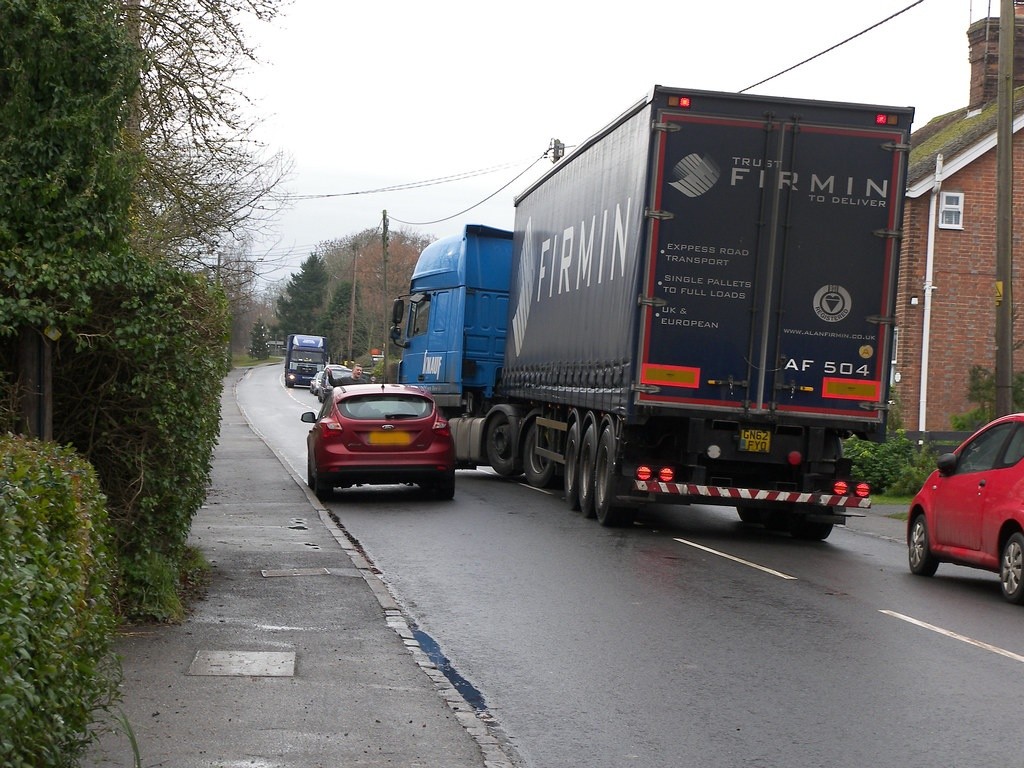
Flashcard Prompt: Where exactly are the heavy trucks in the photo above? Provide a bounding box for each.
[388,81,914,539]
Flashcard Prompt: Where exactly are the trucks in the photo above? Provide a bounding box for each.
[285,334,327,389]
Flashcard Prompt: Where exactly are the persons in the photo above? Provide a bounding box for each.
[325,364,376,412]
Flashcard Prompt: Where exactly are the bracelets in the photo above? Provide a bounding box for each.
[371,381,374,382]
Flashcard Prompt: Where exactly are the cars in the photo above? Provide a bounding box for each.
[317,365,355,404]
[905,414,1024,603]
[300,385,459,502]
[309,371,324,397]
